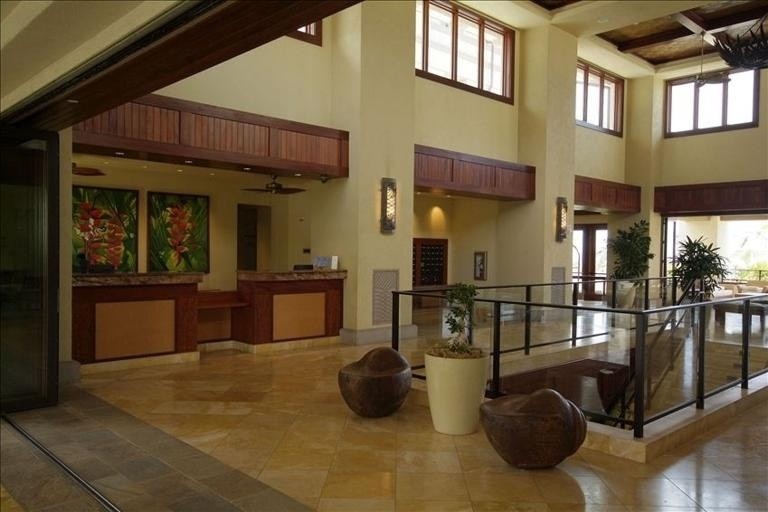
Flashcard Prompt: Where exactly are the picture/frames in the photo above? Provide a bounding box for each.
[145,188,211,275]
[71,183,142,277]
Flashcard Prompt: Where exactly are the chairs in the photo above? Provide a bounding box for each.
[734,279,768,299]
[695,277,738,300]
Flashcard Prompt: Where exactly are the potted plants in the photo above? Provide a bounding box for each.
[607,218,652,308]
[672,235,731,328]
[425,282,492,436]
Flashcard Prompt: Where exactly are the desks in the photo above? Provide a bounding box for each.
[712,301,767,333]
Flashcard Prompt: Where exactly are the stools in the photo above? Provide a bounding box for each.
[479,387,587,471]
[338,346,413,419]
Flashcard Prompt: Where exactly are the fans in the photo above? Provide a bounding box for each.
[239,173,306,197]
[669,28,732,90]
[72,161,103,176]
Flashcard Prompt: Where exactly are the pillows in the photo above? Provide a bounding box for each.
[741,285,758,293]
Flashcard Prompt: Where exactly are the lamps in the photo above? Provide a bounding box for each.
[555,197,569,242]
[378,175,397,230]
[713,0,767,71]
